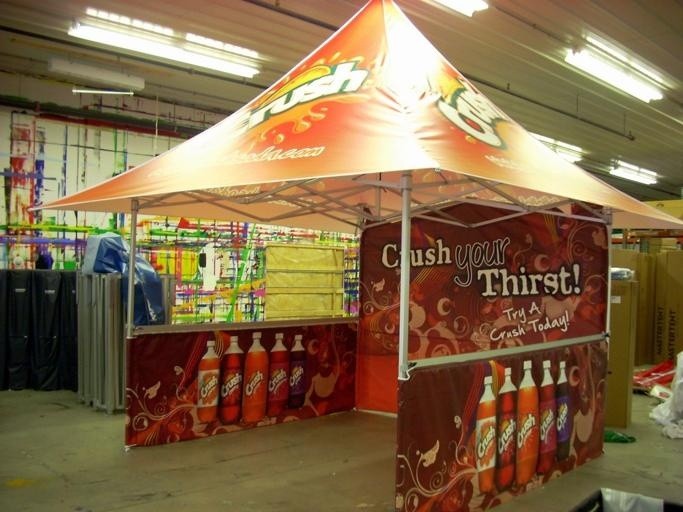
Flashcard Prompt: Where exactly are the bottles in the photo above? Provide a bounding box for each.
[196,331,314,427]
[475,358,571,495]
